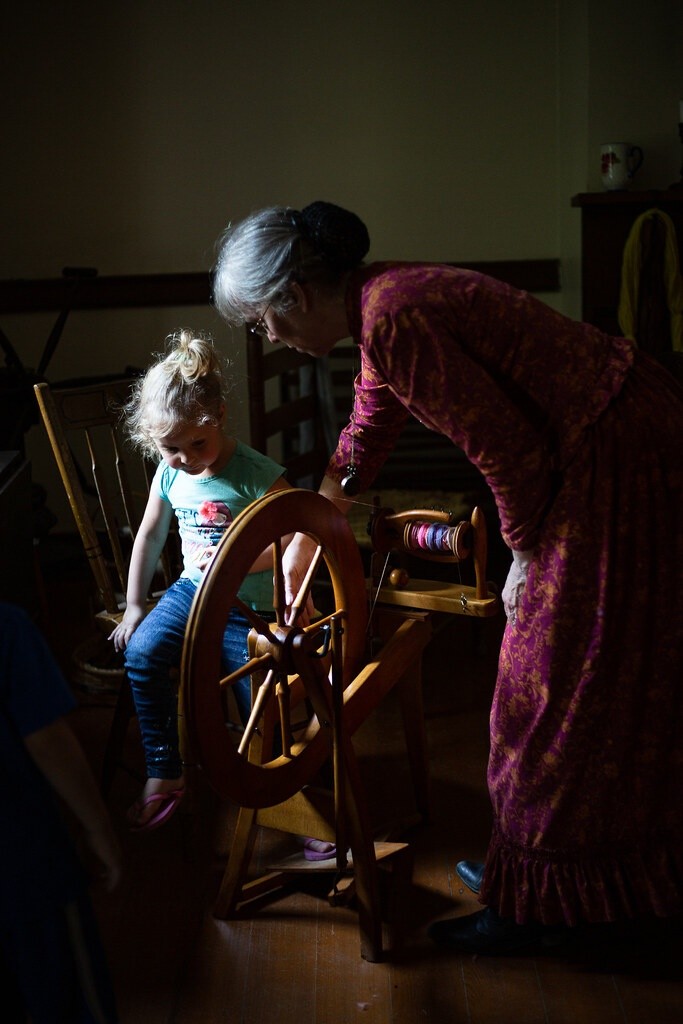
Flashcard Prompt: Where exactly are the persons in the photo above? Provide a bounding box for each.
[218,205,683,958]
[0,599,125,1024]
[106,351,304,830]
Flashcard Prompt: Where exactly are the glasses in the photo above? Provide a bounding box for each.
[249,300,272,338]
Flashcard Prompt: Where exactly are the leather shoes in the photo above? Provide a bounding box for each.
[428,906,544,955]
[456,859,485,893]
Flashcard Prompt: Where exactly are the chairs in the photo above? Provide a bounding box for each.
[27,382,250,839]
[247,318,483,705]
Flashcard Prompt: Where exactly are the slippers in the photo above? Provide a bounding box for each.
[124,780,186,833]
[304,834,337,861]
